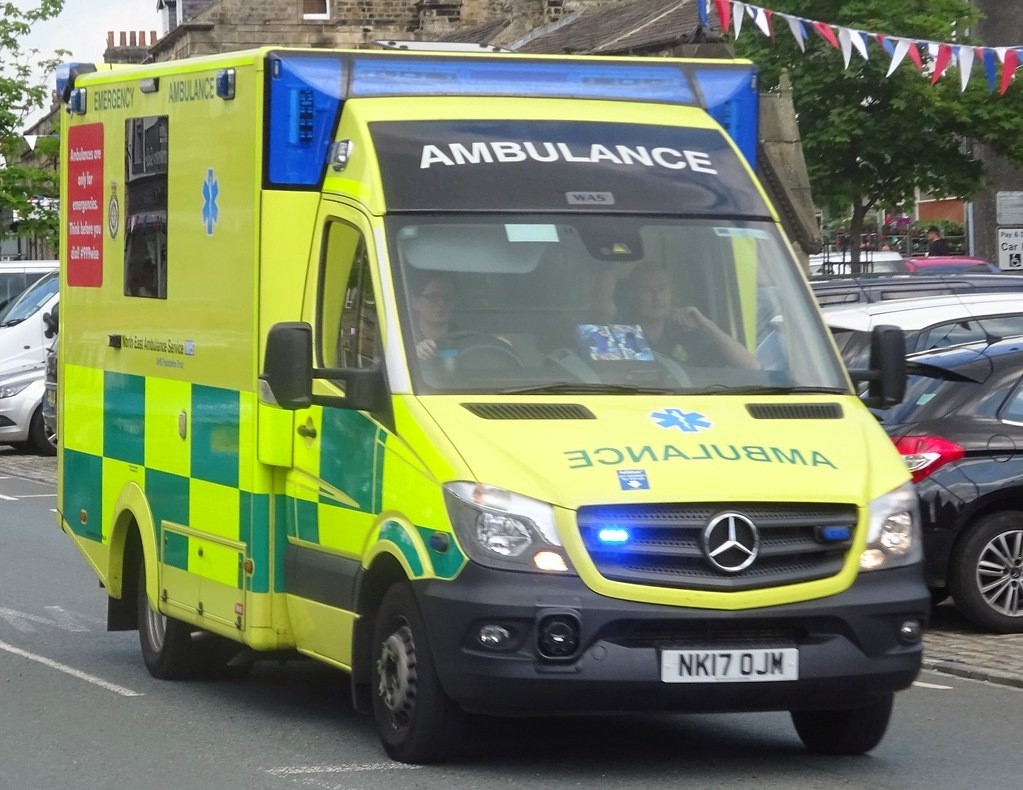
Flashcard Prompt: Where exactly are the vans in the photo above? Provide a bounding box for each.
[0,260,59,457]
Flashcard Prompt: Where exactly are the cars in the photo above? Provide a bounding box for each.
[806,251,1023,634]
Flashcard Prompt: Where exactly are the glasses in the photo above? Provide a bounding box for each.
[421,291,458,304]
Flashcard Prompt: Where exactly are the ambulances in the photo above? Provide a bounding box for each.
[56,45,932,765]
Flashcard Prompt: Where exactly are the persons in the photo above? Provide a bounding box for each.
[926,225,952,256]
[379,267,511,374]
[594,257,764,385]
